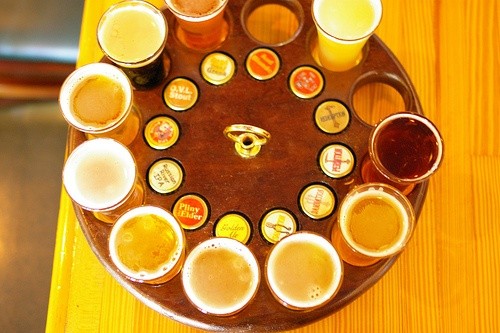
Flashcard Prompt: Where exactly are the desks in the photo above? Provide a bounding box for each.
[43,0,500,333]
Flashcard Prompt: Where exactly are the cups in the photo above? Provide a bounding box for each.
[264,231,345,311]
[164,0,229,50]
[107,204,187,286]
[61,137,142,221]
[311,0,383,71]
[338,183,417,264]
[180,236,261,316]
[97,0,169,89]
[58,63,132,137]
[365,111,444,192]
